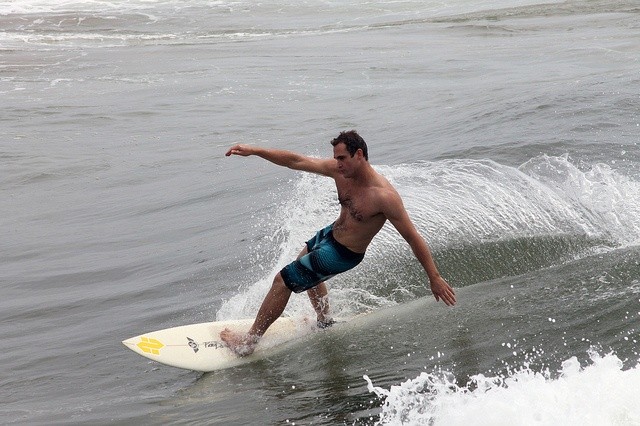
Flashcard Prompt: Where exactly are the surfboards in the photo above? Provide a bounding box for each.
[122,316,340,372]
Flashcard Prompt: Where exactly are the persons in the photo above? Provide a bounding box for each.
[220,130,457,358]
